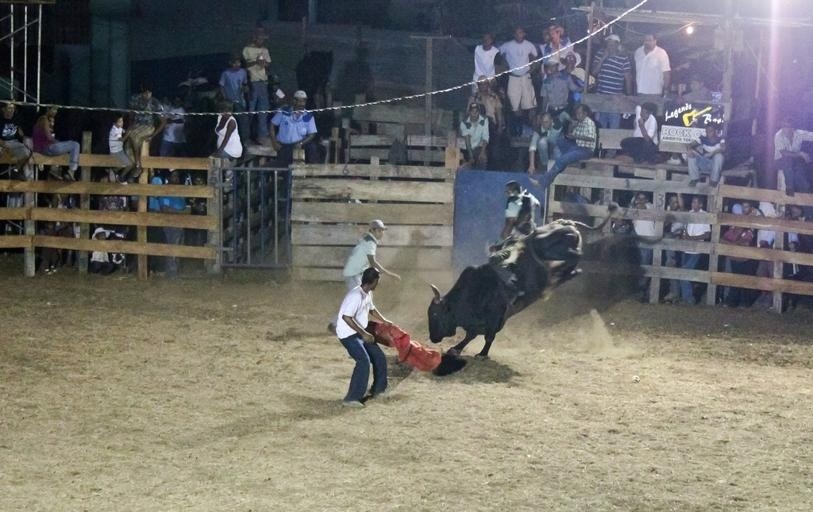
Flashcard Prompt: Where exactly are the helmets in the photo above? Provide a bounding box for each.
[294,90,309,100]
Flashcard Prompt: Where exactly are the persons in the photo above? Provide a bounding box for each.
[459,25,813,309]
[0,22,361,276]
[329,321,465,374]
[335,267,392,408]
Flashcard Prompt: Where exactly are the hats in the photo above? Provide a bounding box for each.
[370,220,388,230]
[91,227,110,239]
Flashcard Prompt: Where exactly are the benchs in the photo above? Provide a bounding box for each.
[573,94,716,182]
